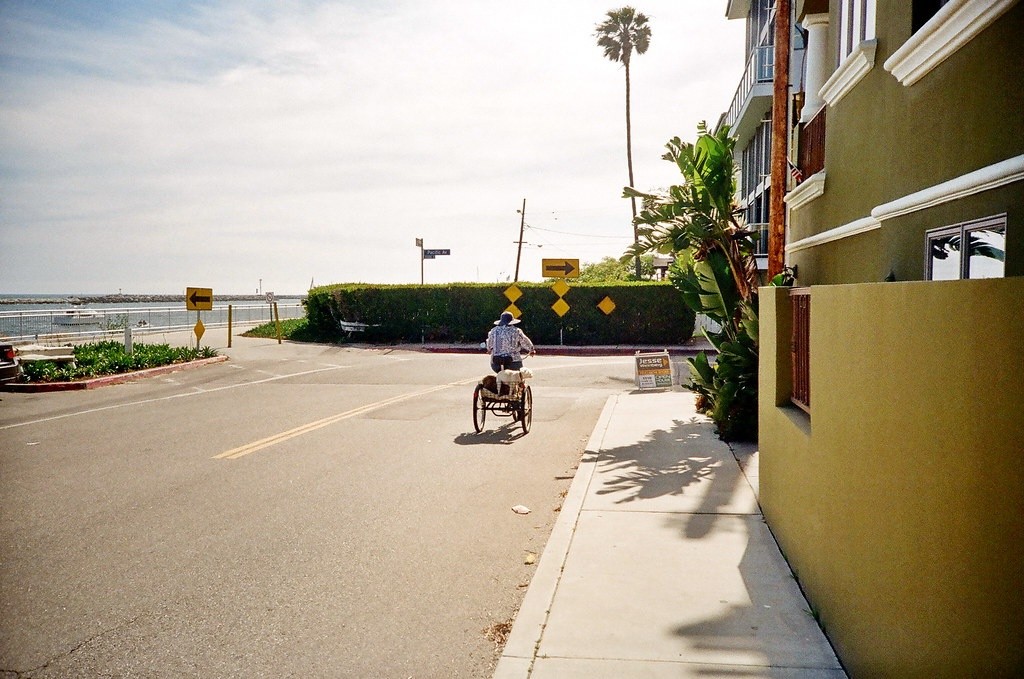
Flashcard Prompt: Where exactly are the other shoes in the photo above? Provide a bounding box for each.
[517,411,522,420]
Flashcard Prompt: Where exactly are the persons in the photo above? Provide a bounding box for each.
[487,311,534,374]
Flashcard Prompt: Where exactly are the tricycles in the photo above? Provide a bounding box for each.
[472,340,533,434]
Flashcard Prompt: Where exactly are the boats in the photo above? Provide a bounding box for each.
[51,305,108,326]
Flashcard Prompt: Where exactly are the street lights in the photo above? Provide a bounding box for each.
[259,279,262,295]
[513,209,525,282]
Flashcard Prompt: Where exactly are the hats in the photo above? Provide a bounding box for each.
[494,312,521,325]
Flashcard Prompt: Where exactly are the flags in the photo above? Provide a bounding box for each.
[787,159,802,183]
